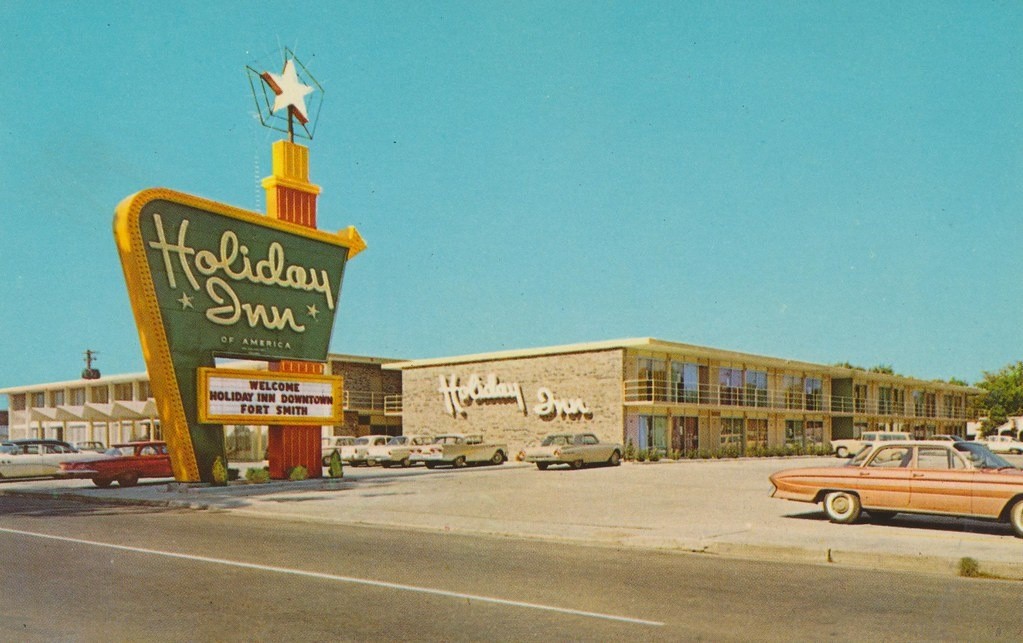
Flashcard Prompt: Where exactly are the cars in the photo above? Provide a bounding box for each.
[920,434,966,457]
[408,432,509,469]
[0,438,175,488]
[987,433,1023,455]
[768,440,1023,538]
[832,430,917,460]
[517,431,624,470]
[320,433,434,469]
